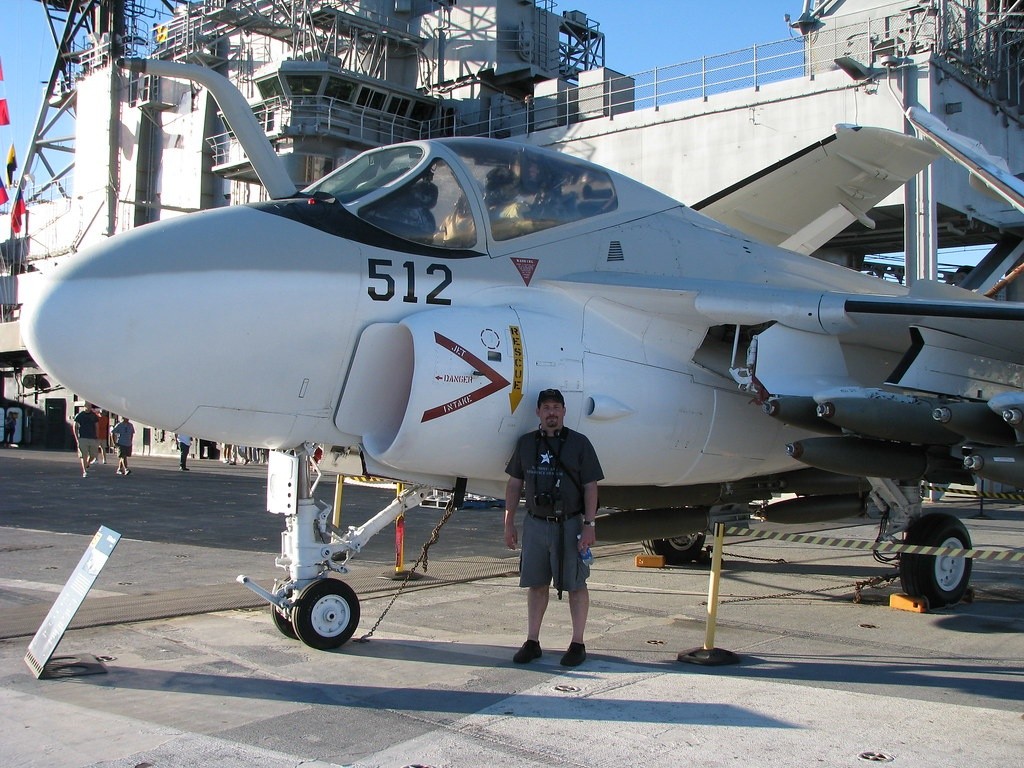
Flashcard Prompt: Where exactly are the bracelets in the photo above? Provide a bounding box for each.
[582,518,596,527]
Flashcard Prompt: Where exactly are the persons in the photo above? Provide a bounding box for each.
[2,411,17,448]
[223,444,269,466]
[110,417,135,476]
[73,400,100,478]
[433,158,570,243]
[90,411,108,464]
[504,388,604,667]
[310,442,330,472]
[175,433,193,471]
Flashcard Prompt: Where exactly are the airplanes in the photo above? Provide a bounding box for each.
[22,57,1024,652]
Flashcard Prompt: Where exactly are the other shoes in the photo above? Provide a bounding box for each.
[513,641,542,663]
[560,644,586,666]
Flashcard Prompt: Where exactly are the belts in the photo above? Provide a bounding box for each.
[528,511,580,522]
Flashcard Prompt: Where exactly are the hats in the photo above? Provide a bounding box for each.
[537,389,565,408]
